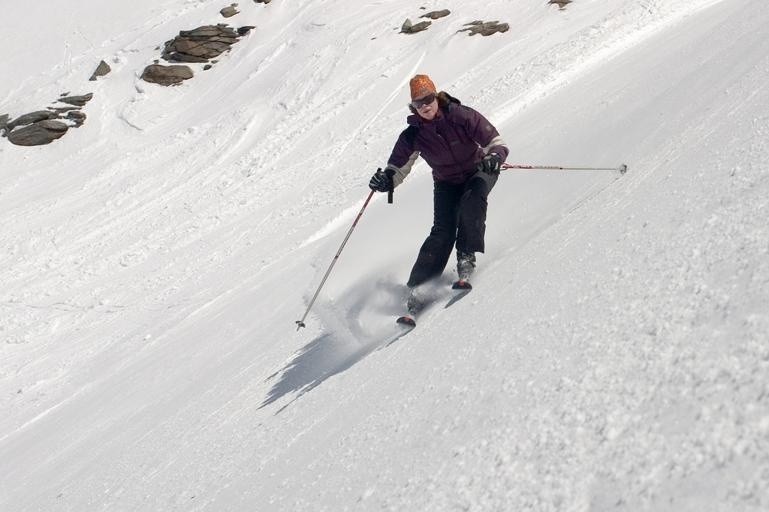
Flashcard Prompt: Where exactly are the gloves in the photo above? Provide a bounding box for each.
[369,172,389,192]
[481,153,504,173]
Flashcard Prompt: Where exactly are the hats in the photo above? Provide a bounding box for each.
[410,75,436,98]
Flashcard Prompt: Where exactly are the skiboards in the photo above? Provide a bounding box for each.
[396,266,472,327]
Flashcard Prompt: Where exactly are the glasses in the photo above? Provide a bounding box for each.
[412,93,434,109]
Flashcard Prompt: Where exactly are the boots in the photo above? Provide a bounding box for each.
[457,249,476,273]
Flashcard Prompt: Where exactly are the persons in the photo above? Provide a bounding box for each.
[369,74,510,316]
